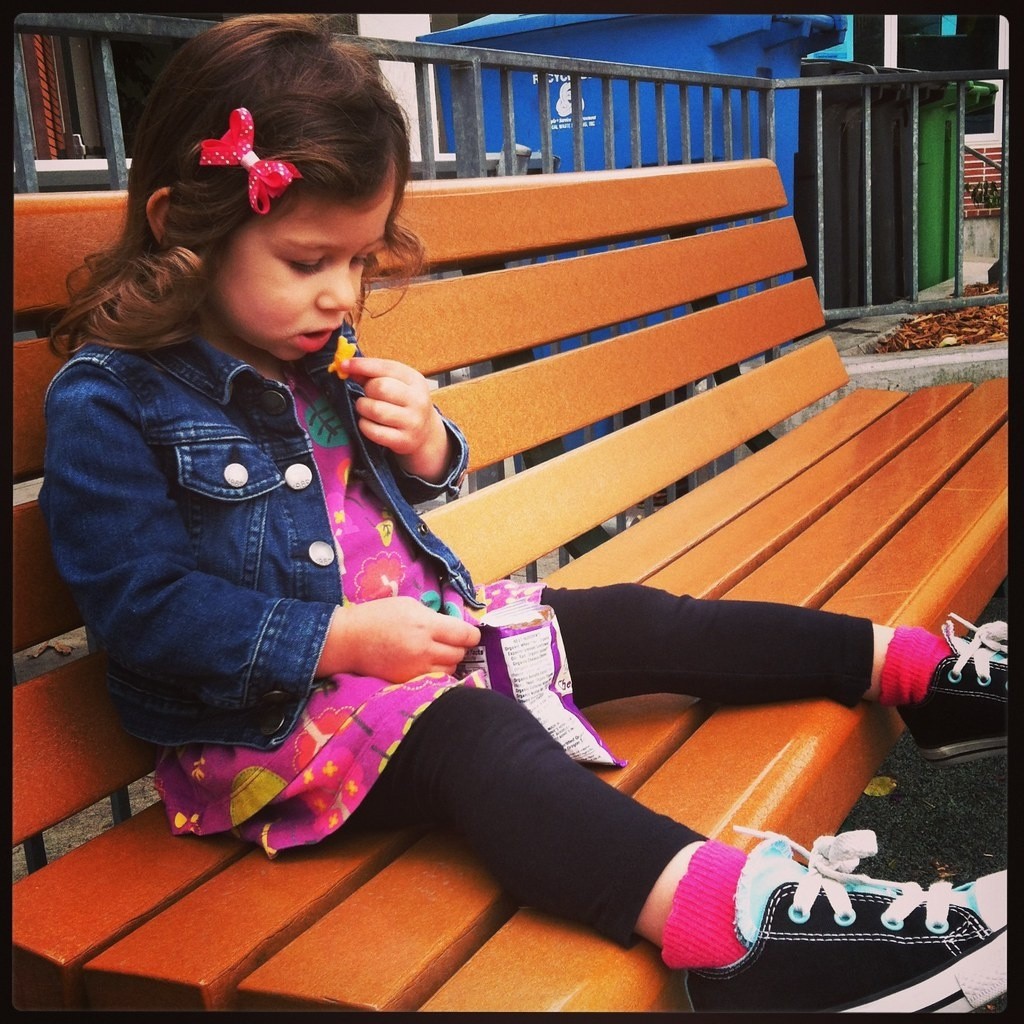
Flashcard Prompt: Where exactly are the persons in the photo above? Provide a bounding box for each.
[35,17,1009,1013]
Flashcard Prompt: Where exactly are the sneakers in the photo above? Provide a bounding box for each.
[897,612,1011,764]
[683,825,1009,1012]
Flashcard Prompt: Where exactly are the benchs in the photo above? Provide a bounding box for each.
[14,158,1007,1013]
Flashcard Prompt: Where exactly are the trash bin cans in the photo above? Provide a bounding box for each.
[795,57,949,344]
[416,12,850,477]
[918,79,999,292]
